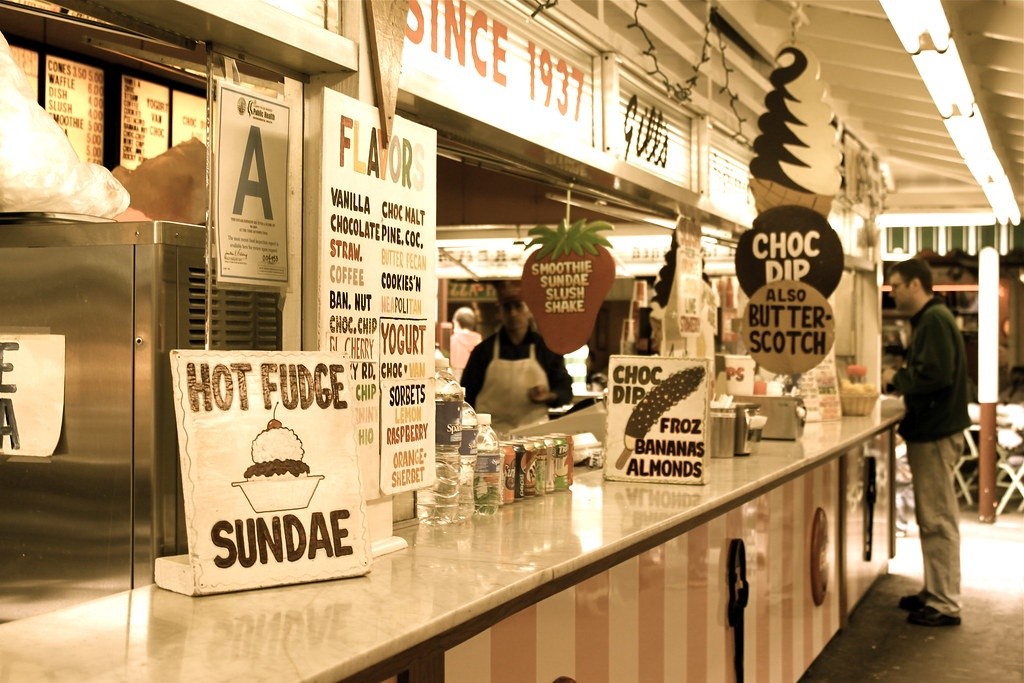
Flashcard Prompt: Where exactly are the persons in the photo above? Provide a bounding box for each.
[883,259,973,627]
[459,286,572,432]
[450,307,482,382]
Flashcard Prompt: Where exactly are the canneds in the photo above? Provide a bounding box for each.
[498,433,575,503]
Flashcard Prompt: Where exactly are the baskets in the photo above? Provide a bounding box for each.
[838,392,878,416]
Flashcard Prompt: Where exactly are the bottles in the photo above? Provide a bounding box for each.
[476,414,501,515]
[459,386,480,520]
[717,276,736,309]
[619,280,654,353]
[415,358,463,527]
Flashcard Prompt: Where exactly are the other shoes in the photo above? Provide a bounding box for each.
[897,589,936,612]
[906,607,961,627]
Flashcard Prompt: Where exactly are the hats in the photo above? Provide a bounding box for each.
[497,286,524,304]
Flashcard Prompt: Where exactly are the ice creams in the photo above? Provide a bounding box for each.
[748,40,843,220]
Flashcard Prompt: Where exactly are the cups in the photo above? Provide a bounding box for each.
[723,354,755,395]
[709,407,735,458]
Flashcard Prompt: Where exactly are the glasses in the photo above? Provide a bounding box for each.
[890,274,918,290]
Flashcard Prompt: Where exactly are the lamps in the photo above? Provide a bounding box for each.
[876,0,1022,225]
